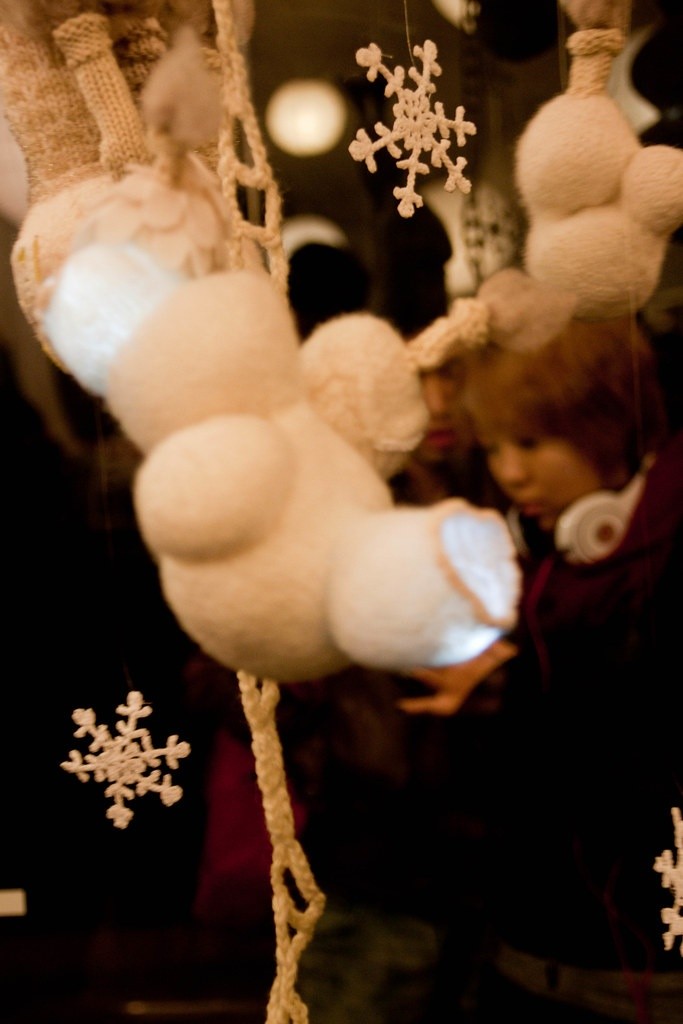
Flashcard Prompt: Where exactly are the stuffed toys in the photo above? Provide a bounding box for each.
[0,0,522,688]
[512,27,682,315]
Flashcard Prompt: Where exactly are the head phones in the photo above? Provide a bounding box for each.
[507,453,655,571]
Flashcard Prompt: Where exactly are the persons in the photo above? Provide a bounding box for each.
[303,314,682,1021]
